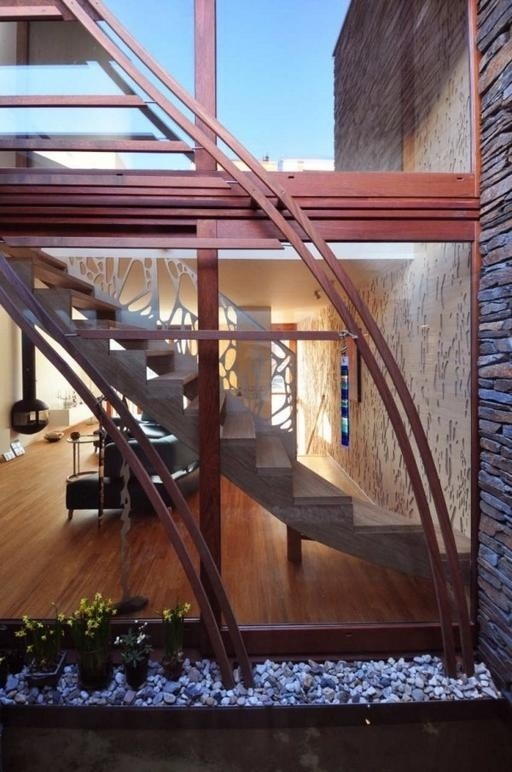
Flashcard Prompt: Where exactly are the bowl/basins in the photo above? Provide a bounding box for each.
[45,432,65,442]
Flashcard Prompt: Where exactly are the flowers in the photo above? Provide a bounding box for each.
[160,597,192,656]
[57,591,118,647]
[111,616,155,668]
[13,601,70,672]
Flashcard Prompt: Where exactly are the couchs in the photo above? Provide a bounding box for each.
[64,413,202,520]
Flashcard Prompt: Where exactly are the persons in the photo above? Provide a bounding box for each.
[263,156,269,161]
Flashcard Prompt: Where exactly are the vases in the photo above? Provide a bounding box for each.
[78,647,114,692]
[164,656,183,681]
[26,651,67,687]
[126,660,148,688]
